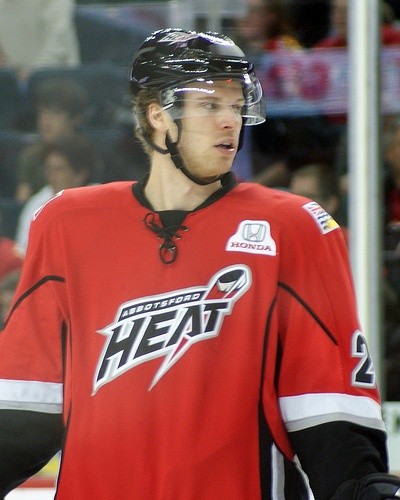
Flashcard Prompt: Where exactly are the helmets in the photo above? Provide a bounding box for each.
[129,29,255,94]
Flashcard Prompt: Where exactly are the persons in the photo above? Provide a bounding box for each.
[0,0,400,402]
[0,27,400,500]
